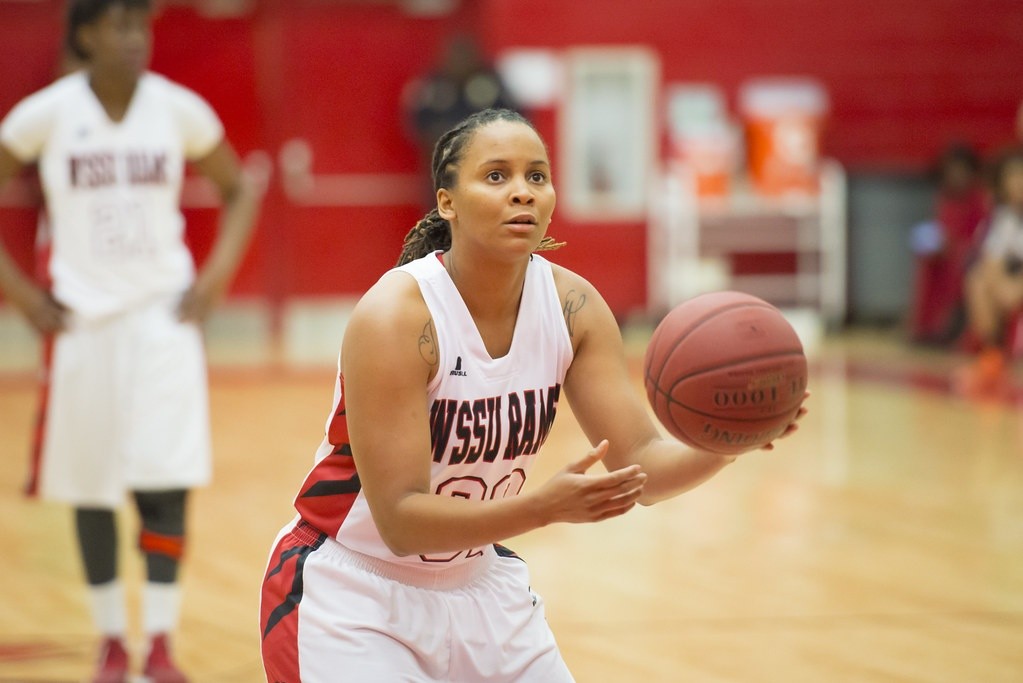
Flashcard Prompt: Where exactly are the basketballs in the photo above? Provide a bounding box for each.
[643,290,809,460]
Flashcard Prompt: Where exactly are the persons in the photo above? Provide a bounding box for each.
[417,43,511,208]
[910,146,1023,392]
[0,0,259,682]
[259,108,809,683]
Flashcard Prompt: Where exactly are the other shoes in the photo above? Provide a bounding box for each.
[94,637,129,683]
[143,635,188,683]
